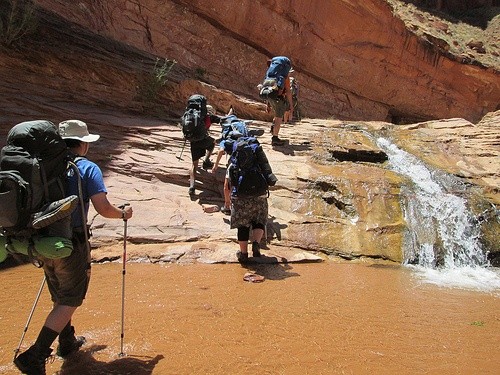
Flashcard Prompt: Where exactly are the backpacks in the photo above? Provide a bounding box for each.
[256,55,291,99]
[220,115,249,155]
[179,93,208,139]
[228,136,277,199]
[0,119,85,263]
[288,76,295,95]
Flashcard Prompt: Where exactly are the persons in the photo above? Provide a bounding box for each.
[188,104,233,197]
[224,157,268,264]
[265,66,294,145]
[282,67,297,124]
[212,132,238,213]
[11,120,133,375]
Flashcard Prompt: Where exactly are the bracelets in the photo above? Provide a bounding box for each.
[121,209,125,219]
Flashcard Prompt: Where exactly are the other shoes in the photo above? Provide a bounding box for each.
[32,195,79,230]
[272,137,285,146]
[221,206,231,215]
[56,335,86,357]
[203,160,214,169]
[189,188,195,196]
[13,345,48,375]
[252,241,261,257]
[236,249,249,267]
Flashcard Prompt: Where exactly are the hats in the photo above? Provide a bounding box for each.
[206,104,215,115]
[58,119,99,144]
